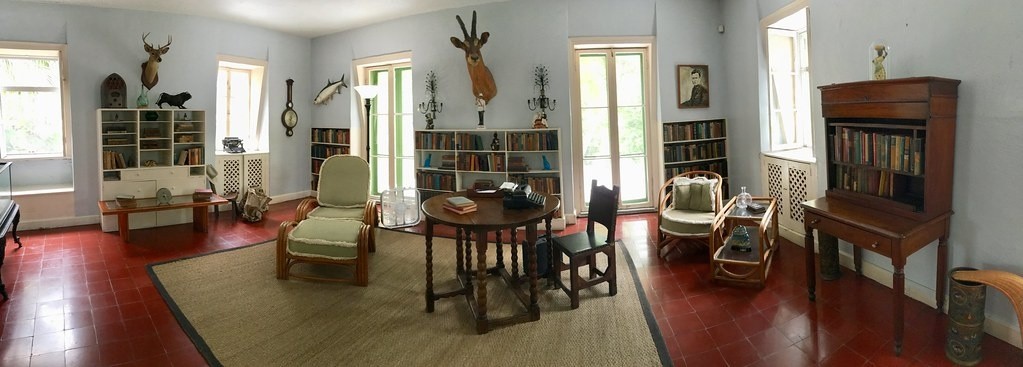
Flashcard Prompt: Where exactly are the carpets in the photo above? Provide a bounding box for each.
[145,225,675,367]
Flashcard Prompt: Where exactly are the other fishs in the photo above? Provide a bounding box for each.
[314,73,347,106]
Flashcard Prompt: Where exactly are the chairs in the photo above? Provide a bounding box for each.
[657,170,724,257]
[553,179,619,308]
[294,155,379,228]
[209,181,238,223]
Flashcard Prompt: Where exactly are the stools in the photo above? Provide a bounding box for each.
[276,220,376,287]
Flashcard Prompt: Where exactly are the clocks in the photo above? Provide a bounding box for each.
[282,79,298,136]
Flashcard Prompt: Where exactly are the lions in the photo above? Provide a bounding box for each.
[156,91,192,109]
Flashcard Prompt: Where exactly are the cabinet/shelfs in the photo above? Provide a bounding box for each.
[415,128,566,230]
[96,109,207,233]
[799,196,954,357]
[710,196,779,288]
[800,76,961,223]
[663,119,730,207]
[310,128,350,198]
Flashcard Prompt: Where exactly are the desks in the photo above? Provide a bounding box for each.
[98,193,229,241]
[421,191,560,334]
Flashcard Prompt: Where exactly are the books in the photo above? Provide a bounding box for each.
[312,129,350,159]
[438,153,529,173]
[507,131,558,151]
[193,188,213,202]
[116,195,137,208]
[143,140,160,149]
[443,197,478,214]
[663,124,729,207]
[103,150,126,169]
[144,128,161,138]
[746,203,766,214]
[417,171,455,205]
[107,127,127,133]
[416,132,484,150]
[836,164,906,200]
[828,125,925,175]
[107,138,128,145]
[508,174,560,194]
[177,146,204,165]
[311,160,324,191]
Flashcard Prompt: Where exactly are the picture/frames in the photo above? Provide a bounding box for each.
[678,65,710,109]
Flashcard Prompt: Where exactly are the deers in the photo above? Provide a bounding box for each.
[142,30,173,91]
[452,11,502,102]
